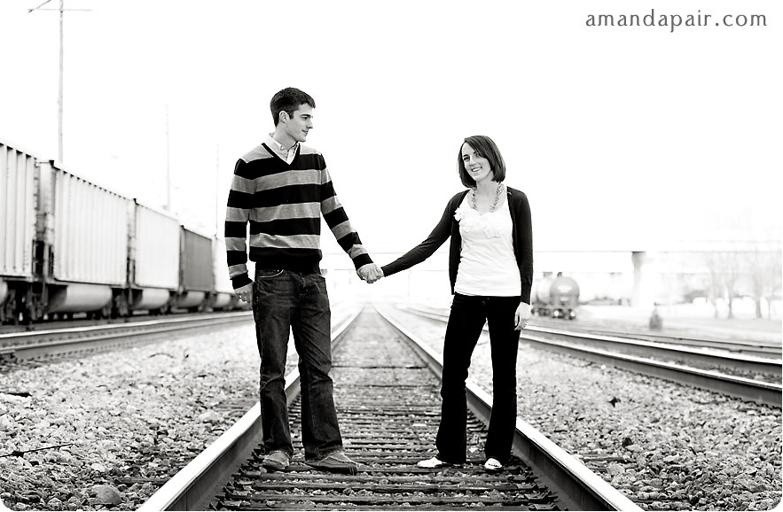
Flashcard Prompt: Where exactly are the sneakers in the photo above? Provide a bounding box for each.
[484,456,504,473]
[304,450,358,475]
[416,455,464,469]
[262,448,292,472]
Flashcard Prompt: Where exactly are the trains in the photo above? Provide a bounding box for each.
[532,270,578,319]
[0,142,249,321]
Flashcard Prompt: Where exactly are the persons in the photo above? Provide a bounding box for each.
[355,135,534,473]
[224,86,383,471]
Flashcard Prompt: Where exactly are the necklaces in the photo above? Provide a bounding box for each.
[471,181,503,213]
